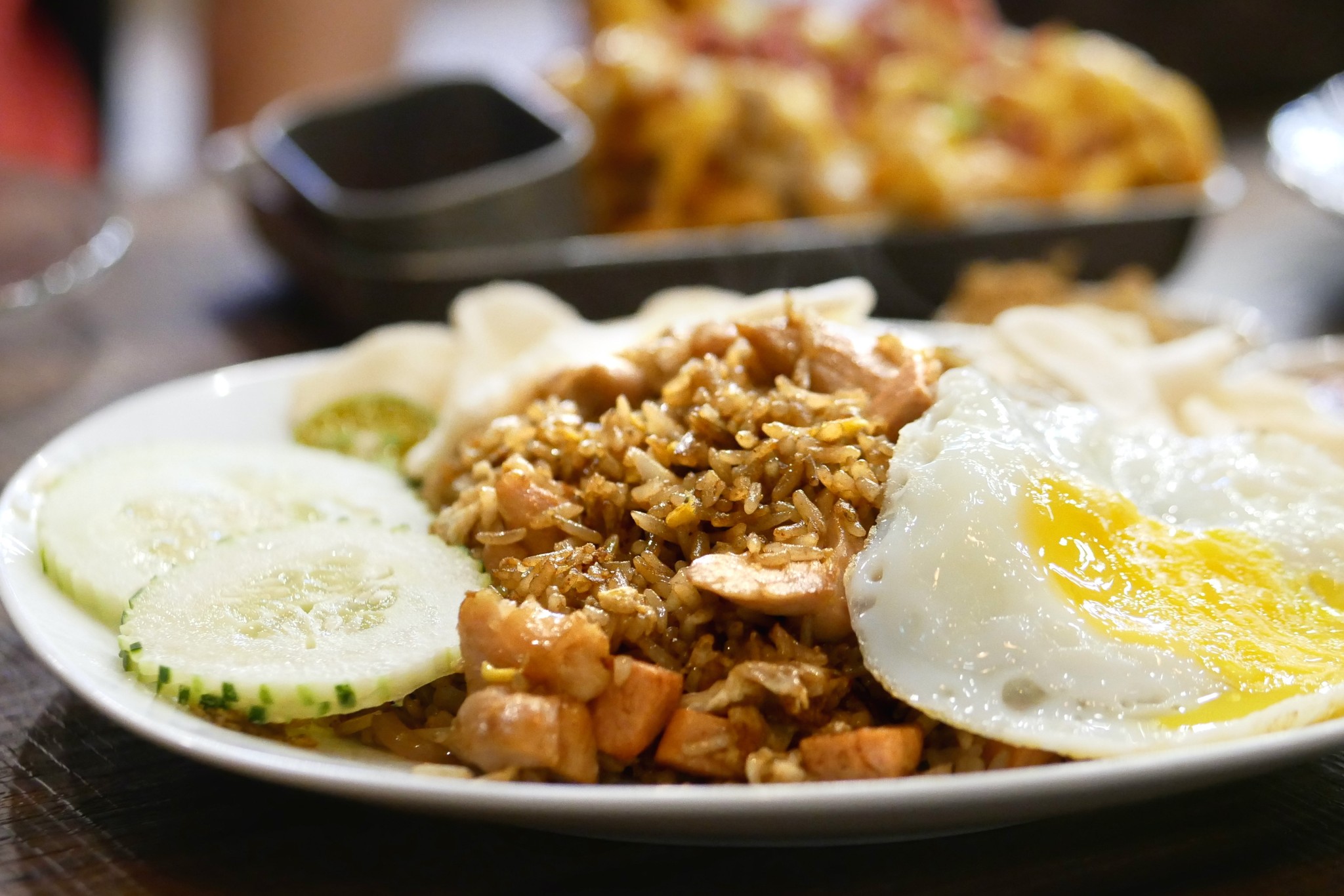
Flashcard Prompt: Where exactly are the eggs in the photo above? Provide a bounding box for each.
[842,300,1344,759]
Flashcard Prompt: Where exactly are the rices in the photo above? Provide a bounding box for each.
[427,290,1066,786]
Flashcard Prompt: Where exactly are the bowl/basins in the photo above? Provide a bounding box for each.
[250,60,597,249]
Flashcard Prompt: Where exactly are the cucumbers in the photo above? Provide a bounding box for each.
[39,439,488,717]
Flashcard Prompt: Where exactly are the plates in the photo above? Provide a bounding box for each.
[0,316,1341,844]
[192,83,1248,316]
[1265,63,1343,239]
